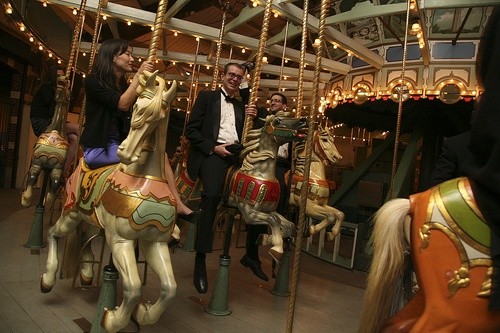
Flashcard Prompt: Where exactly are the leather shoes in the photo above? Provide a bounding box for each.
[240,253,270,282]
[192,252,209,294]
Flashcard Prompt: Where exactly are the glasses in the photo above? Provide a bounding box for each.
[226,73,244,81]
[269,98,285,105]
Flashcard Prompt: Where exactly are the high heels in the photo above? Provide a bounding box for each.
[177,206,202,224]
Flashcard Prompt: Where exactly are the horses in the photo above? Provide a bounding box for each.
[210,113,305,263]
[360,178,500,333]
[287,124,345,240]
[42,70,177,333]
[21,74,71,212]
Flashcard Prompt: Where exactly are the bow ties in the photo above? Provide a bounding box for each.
[219,87,238,104]
[267,110,277,115]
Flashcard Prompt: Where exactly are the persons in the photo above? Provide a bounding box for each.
[79,39,204,245]
[185,62,270,294]
[30,65,80,160]
[470,5,500,313]
[256,93,293,216]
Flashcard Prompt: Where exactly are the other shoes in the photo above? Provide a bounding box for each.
[282,237,293,252]
